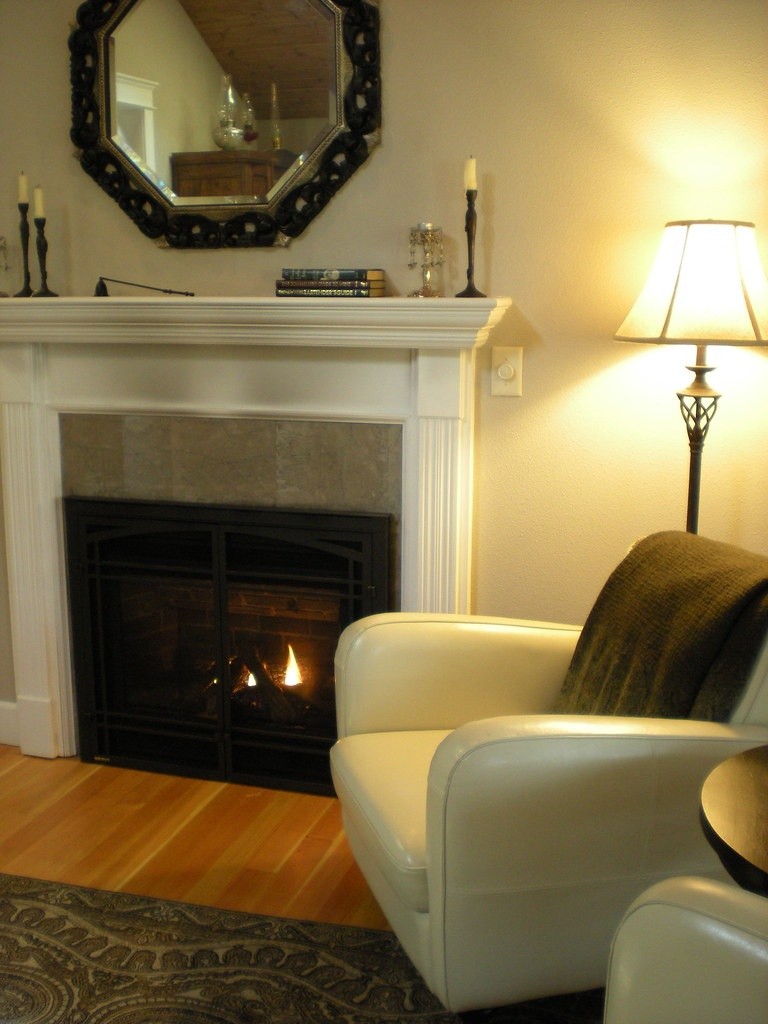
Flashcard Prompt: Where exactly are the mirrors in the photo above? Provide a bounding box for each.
[68,1,384,251]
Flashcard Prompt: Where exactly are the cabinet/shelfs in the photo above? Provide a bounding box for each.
[169,148,297,198]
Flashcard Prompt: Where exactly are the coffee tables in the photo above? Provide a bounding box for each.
[694,745,766,894]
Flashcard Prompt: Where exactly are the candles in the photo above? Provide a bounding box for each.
[15,167,29,203]
[32,181,46,218]
[462,153,480,192]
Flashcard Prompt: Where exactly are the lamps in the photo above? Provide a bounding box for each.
[610,217,765,533]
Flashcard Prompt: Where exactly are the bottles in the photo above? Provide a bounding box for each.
[213,73,245,151]
[240,90,260,151]
[269,83,285,152]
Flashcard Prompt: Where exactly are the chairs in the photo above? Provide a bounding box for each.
[325,528,767,1010]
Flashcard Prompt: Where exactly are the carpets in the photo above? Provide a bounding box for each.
[0,870,466,1023]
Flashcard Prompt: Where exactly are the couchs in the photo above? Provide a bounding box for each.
[598,878,767,1024]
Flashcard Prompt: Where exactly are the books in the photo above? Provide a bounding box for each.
[274,266,386,299]
